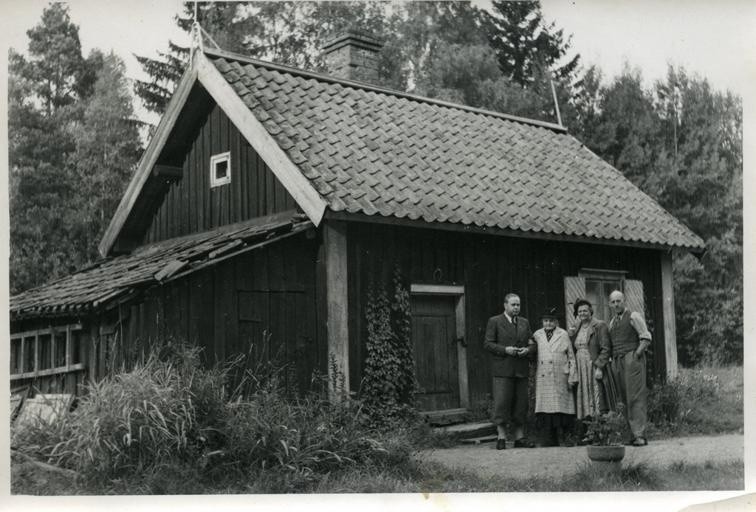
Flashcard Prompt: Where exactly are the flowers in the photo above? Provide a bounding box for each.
[581,401,629,445]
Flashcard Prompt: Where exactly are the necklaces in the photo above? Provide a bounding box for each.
[581,322,590,331]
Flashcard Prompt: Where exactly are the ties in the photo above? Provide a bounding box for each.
[512,317,517,326]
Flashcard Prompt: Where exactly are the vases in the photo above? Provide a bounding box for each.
[589,446,625,478]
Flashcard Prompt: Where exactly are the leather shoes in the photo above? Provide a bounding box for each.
[496,439,505,450]
[624,438,646,445]
[515,437,535,448]
[540,433,593,447]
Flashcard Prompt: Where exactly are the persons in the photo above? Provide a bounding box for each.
[563,299,611,446]
[595,290,653,446]
[483,292,536,450]
[528,305,578,447]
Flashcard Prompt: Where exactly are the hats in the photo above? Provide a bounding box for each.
[539,304,561,321]
